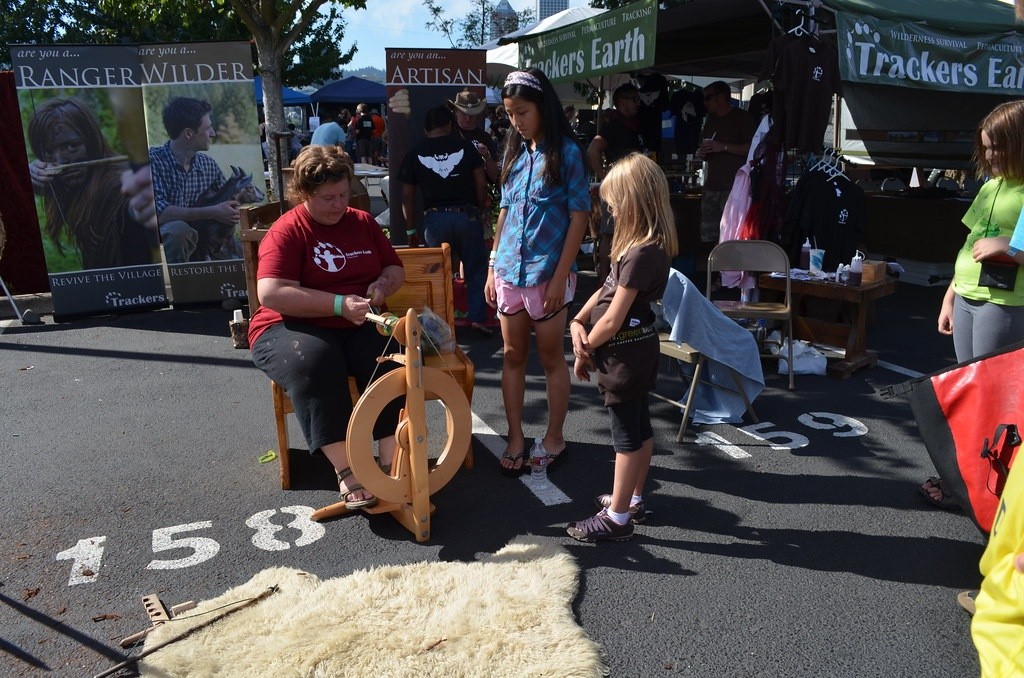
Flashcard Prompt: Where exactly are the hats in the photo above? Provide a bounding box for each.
[339,108,350,117]
[369,108,381,115]
[357,102,368,114]
[447,91,488,116]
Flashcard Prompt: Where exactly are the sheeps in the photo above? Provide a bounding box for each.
[188,165,266,263]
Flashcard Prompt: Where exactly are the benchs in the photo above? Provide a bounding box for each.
[273,242,475,490]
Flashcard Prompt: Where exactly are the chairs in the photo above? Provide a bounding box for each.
[653,239,794,443]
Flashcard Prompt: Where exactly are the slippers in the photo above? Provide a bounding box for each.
[523,444,568,474]
[498,449,525,477]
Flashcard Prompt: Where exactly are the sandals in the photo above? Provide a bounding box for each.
[920,479,960,506]
[337,467,378,510]
[377,458,440,516]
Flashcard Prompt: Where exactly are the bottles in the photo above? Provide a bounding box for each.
[835,263,844,282]
[850,250,866,285]
[529,438,547,490]
[771,330,826,373]
[671,153,693,188]
[757,318,766,341]
[800,237,811,270]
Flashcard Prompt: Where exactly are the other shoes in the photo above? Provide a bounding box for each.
[471,322,495,338]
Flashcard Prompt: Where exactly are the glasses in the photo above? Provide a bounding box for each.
[620,96,641,103]
[701,91,723,103]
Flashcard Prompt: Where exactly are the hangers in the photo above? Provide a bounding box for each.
[810,147,852,183]
[788,8,825,43]
[749,111,774,169]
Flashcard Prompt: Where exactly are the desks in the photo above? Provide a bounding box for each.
[757,269,897,382]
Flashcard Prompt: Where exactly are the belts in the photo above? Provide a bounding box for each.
[422,206,471,213]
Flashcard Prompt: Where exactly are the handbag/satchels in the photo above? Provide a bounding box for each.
[977,259,1019,292]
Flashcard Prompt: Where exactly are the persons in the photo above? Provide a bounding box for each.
[149,97,241,262]
[563,80,758,295]
[249,144,406,509]
[28,95,158,270]
[918,101,1024,678]
[399,92,512,336]
[483,68,592,475]
[259,103,390,167]
[566,151,678,543]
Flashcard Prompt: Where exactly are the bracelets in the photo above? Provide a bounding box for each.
[488,261,494,266]
[406,228,416,235]
[333,295,343,316]
[569,319,584,326]
[489,250,496,259]
[1007,247,1019,257]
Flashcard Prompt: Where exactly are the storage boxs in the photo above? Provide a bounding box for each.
[866,191,975,288]
[863,261,887,283]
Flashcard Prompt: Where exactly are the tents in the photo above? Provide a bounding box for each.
[255,75,315,115]
[310,76,387,103]
[510,0,1024,167]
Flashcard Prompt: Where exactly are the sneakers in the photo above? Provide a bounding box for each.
[567,509,634,542]
[594,492,647,523]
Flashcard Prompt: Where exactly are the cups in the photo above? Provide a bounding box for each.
[809,249,826,275]
[234,309,242,323]
[702,139,713,145]
